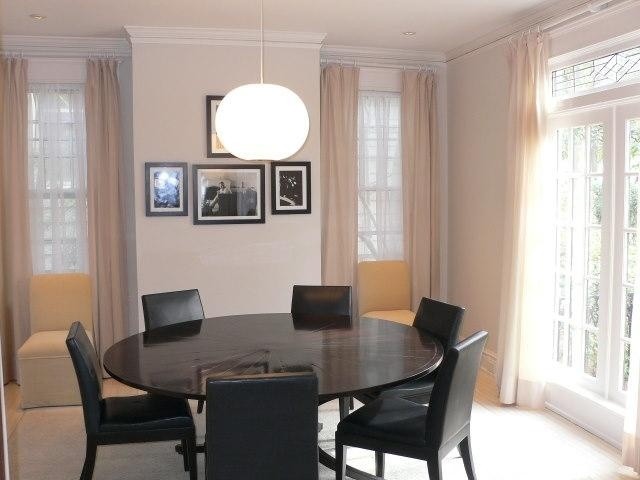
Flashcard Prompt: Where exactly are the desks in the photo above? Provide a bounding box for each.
[103,314,444,480]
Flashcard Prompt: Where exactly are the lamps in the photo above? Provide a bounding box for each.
[215,0,309,161]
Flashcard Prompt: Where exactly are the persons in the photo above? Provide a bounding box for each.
[217,182,232,194]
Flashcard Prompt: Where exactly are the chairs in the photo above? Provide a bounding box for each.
[291,285,354,424]
[66,321,197,480]
[206,372,318,480]
[358,261,416,327]
[142,289,206,413]
[335,329,488,480]
[349,297,469,410]
[17,273,93,409]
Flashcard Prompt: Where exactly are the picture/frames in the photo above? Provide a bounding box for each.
[271,162,311,215]
[145,162,188,216]
[206,95,237,158]
[192,164,265,225]
